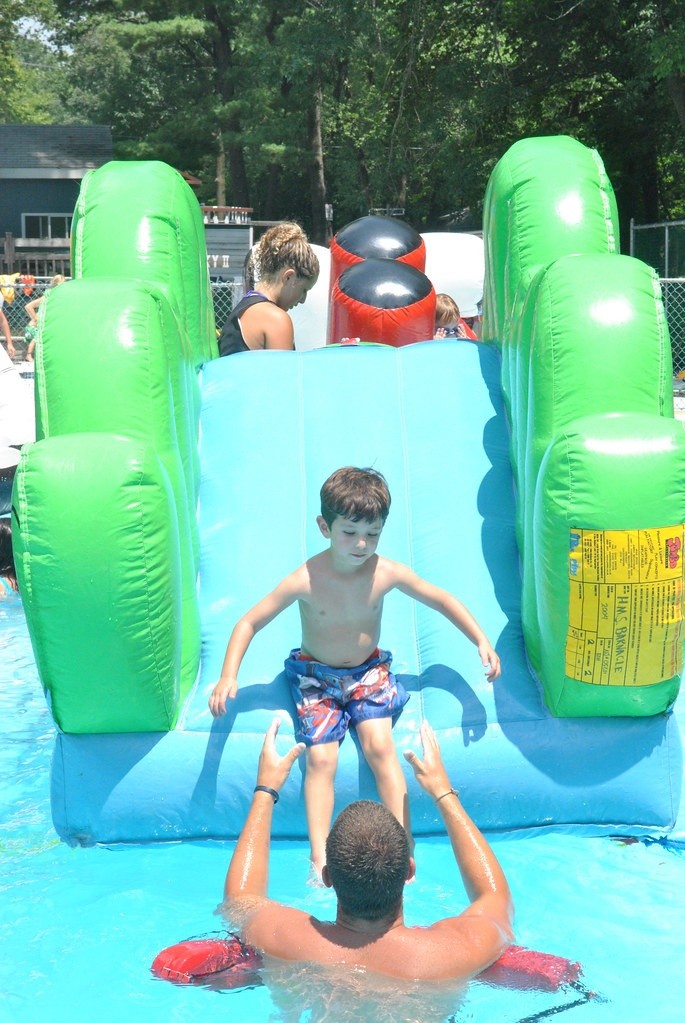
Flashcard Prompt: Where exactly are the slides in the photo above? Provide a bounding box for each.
[9,340,685,854]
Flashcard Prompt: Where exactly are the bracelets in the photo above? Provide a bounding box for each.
[254,786,280,804]
[433,788,457,803]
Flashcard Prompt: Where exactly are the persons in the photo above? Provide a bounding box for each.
[218,222,320,358]
[208,465,502,886]
[225,716,516,987]
[0,275,65,362]
[432,294,472,342]
